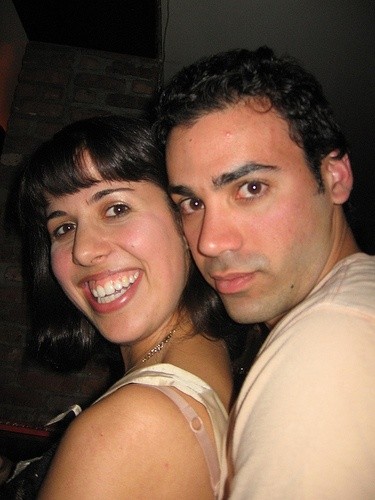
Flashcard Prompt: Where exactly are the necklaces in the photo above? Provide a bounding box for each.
[123,324,179,374]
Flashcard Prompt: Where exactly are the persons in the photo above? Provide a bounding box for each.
[4,120,247,500]
[150,43,375,500]
